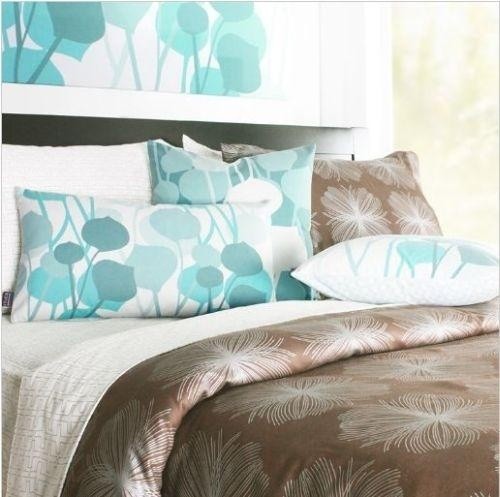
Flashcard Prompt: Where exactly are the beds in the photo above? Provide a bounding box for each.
[2,296,499,497]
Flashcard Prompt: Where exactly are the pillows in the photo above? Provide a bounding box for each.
[290,232,499,306]
[146,138,319,302]
[9,184,277,325]
[219,140,444,257]
